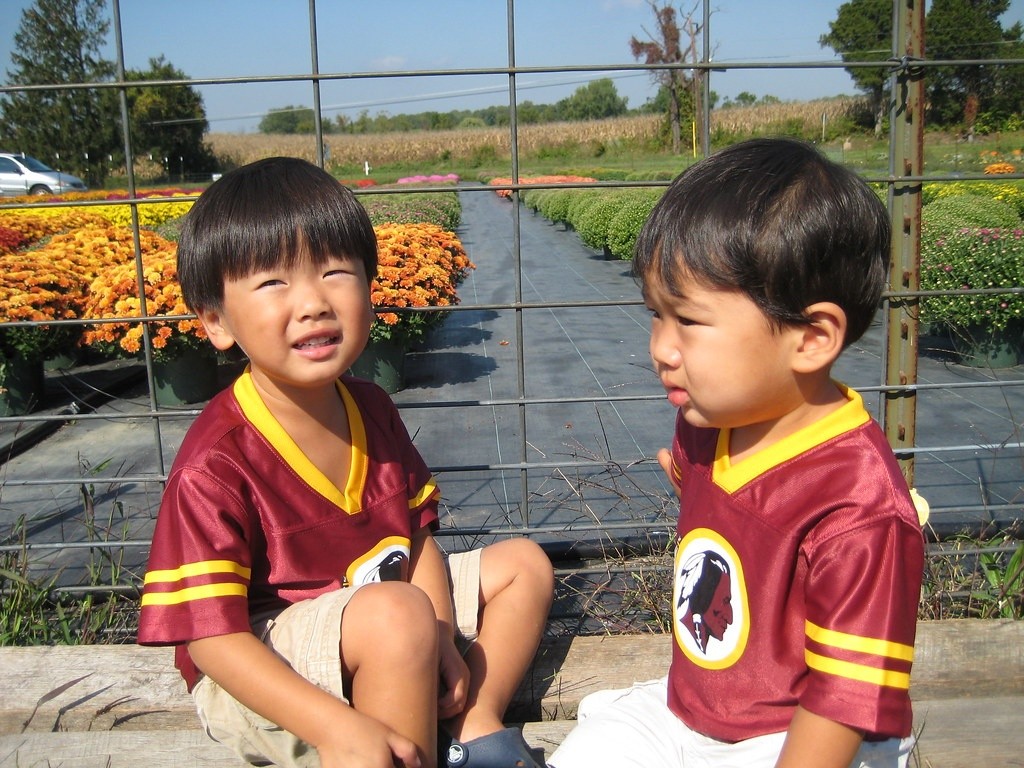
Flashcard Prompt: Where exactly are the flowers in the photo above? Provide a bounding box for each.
[0,175,478,397]
[477,170,1024,335]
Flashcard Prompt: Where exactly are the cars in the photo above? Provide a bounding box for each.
[0,152,91,197]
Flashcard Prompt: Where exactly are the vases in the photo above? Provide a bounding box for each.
[603,245,621,260]
[352,336,405,395]
[951,332,1020,369]
[919,322,947,337]
[43,347,83,370]
[533,207,544,217]
[0,354,49,418]
[565,222,575,233]
[150,346,218,406]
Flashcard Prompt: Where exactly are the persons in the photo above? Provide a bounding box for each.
[137,156,554,768]
[543,138,926,768]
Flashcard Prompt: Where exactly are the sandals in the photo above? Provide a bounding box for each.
[438,724,542,768]
